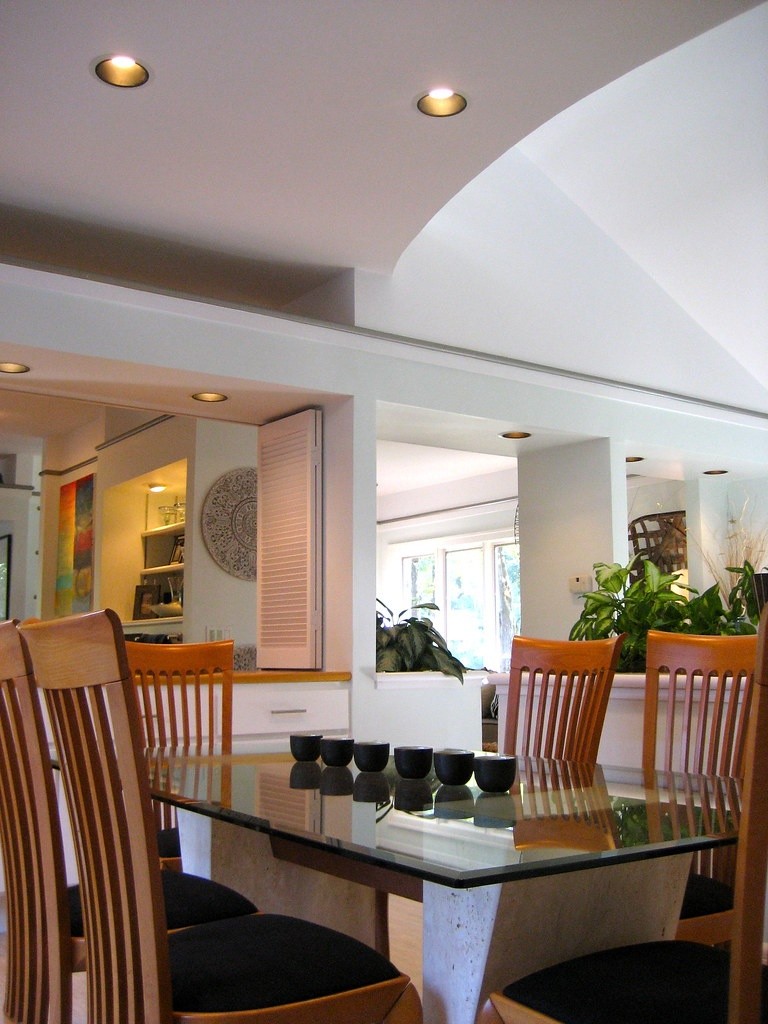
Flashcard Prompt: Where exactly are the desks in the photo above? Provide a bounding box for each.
[40,754,741,1024]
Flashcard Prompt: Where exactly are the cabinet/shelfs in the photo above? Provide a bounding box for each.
[141,520,184,575]
[35,681,349,888]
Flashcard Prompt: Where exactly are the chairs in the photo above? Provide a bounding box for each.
[503,633,628,762]
[15,609,424,1024]
[126,640,234,868]
[488,602,767,1024]
[0,618,262,1024]
[641,629,758,778]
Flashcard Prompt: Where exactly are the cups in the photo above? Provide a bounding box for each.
[167,575,184,604]
[320,766,354,796]
[289,761,322,789]
[434,785,475,819]
[394,745,434,781]
[434,749,475,785]
[353,741,390,772]
[290,735,323,762]
[473,792,517,829]
[394,779,433,811]
[320,738,354,767]
[472,755,516,793]
[353,772,390,803]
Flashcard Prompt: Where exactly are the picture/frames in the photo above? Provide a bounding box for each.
[133,584,161,620]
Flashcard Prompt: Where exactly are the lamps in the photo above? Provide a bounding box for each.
[150,484,167,493]
